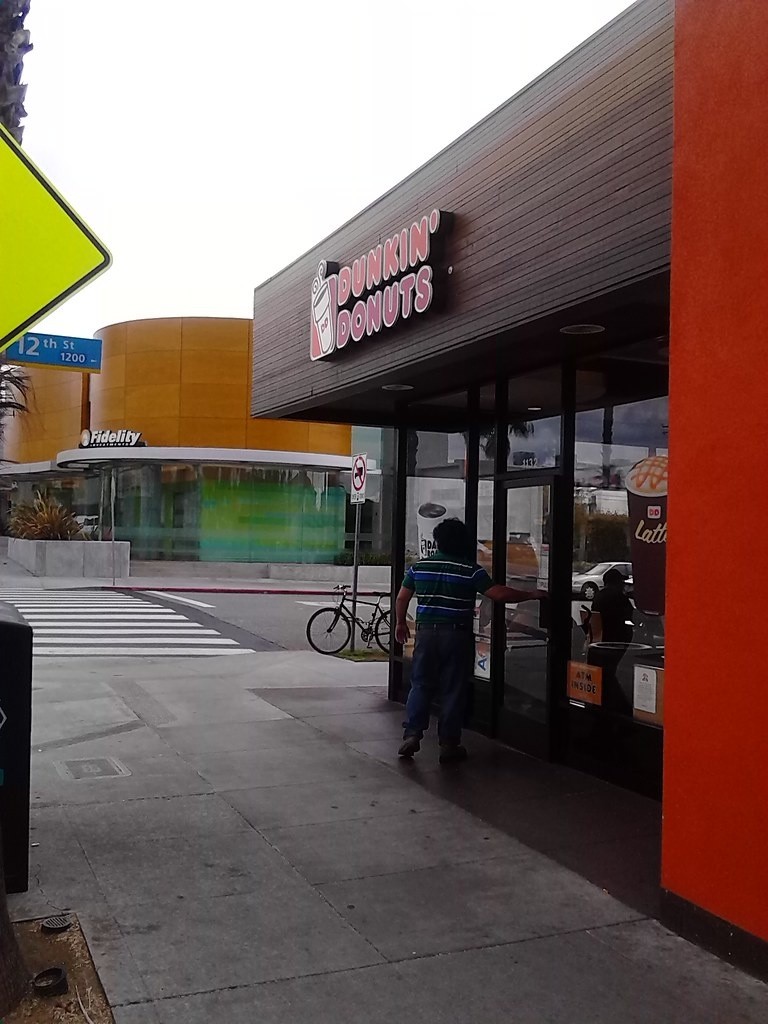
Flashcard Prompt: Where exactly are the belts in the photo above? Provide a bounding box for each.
[418,623,464,629]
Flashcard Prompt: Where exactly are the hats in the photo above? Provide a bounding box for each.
[603,568,629,584]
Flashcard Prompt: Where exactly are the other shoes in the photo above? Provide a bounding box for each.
[439,744,467,764]
[398,735,421,757]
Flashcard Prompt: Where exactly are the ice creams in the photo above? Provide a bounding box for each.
[625,456,669,616]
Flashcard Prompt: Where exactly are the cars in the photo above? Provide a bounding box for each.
[571,562,632,600]
[77,515,100,532]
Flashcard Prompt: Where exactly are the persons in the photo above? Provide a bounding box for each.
[395,518,548,763]
[591,570,634,644]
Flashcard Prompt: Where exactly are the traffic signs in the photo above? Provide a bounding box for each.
[1,332,103,374]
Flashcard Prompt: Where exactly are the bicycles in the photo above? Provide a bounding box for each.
[306,584,414,655]
